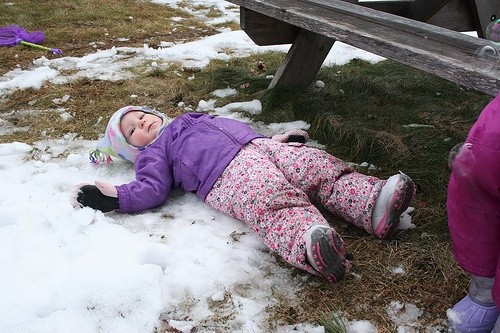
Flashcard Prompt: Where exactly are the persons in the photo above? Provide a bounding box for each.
[69,105,417,282]
[445,91,500,333]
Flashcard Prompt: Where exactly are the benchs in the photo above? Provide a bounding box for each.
[227,0,500,98]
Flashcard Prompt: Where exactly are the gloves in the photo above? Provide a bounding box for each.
[71,180,120,213]
[271,129,308,146]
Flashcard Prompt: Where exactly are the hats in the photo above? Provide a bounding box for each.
[88,106,171,166]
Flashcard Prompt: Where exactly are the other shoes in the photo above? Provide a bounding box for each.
[446,293,500,333]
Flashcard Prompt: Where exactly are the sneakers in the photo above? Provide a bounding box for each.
[371,174,414,240]
[305,225,350,283]
